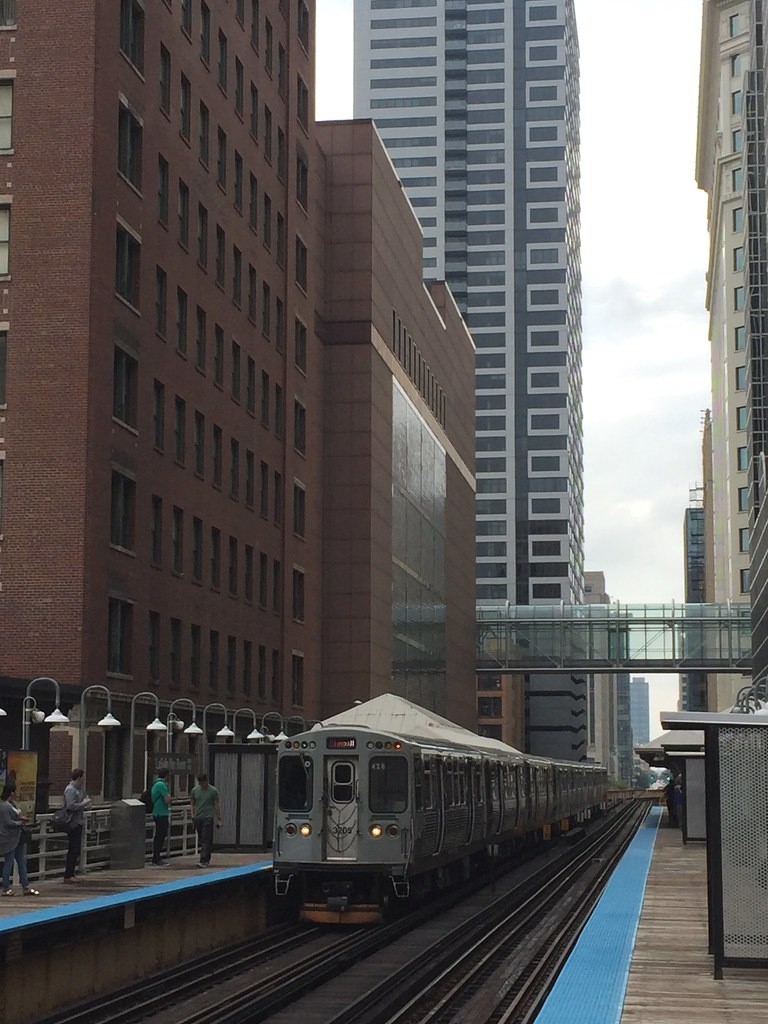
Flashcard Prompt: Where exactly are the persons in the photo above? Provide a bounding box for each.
[152,768,173,867]
[664,779,683,819]
[62,768,93,884]
[190,772,223,868]
[0,783,40,896]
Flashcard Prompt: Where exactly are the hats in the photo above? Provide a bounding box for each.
[197,773,208,782]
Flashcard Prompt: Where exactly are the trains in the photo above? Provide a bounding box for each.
[273,725,607,926]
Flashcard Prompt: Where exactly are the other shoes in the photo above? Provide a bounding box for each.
[2,888,19,896]
[151,860,170,867]
[195,861,210,867]
[63,876,85,883]
[23,886,39,896]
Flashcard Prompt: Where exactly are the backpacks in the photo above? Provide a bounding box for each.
[139,780,165,813]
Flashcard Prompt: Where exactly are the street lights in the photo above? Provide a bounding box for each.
[260,711,290,744]
[127,691,168,799]
[169,698,204,798]
[203,703,236,778]
[79,684,122,874]
[24,676,69,749]
[232,707,265,745]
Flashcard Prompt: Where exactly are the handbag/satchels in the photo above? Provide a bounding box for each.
[50,805,78,831]
[20,819,32,843]
